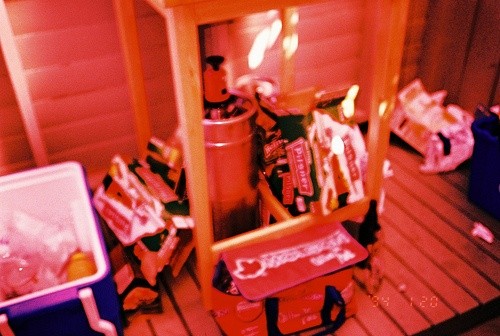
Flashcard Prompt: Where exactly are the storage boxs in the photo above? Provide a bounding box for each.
[0,161,129,335]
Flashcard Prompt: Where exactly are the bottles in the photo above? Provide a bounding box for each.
[2,259,36,296]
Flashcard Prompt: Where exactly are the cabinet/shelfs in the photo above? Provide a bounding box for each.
[113,0,409,294]
